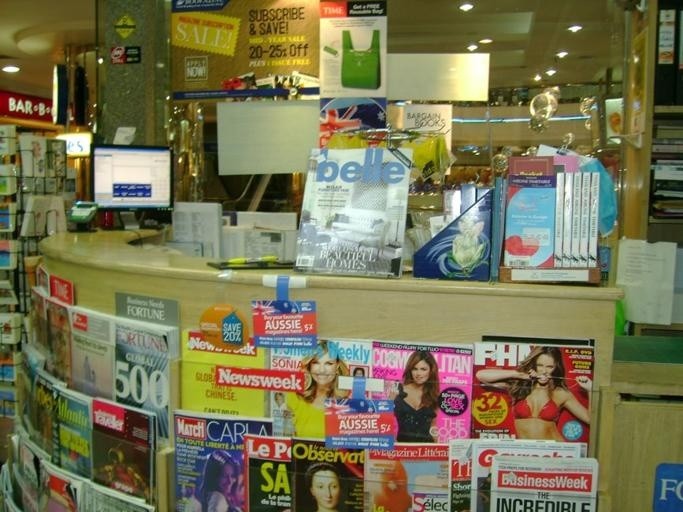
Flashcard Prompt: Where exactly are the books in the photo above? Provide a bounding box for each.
[0,122,601,512]
[649,0,683,224]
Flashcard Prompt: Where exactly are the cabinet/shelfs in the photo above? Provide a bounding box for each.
[620,16,682,337]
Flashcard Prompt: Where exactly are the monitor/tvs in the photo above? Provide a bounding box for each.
[90,142,175,231]
